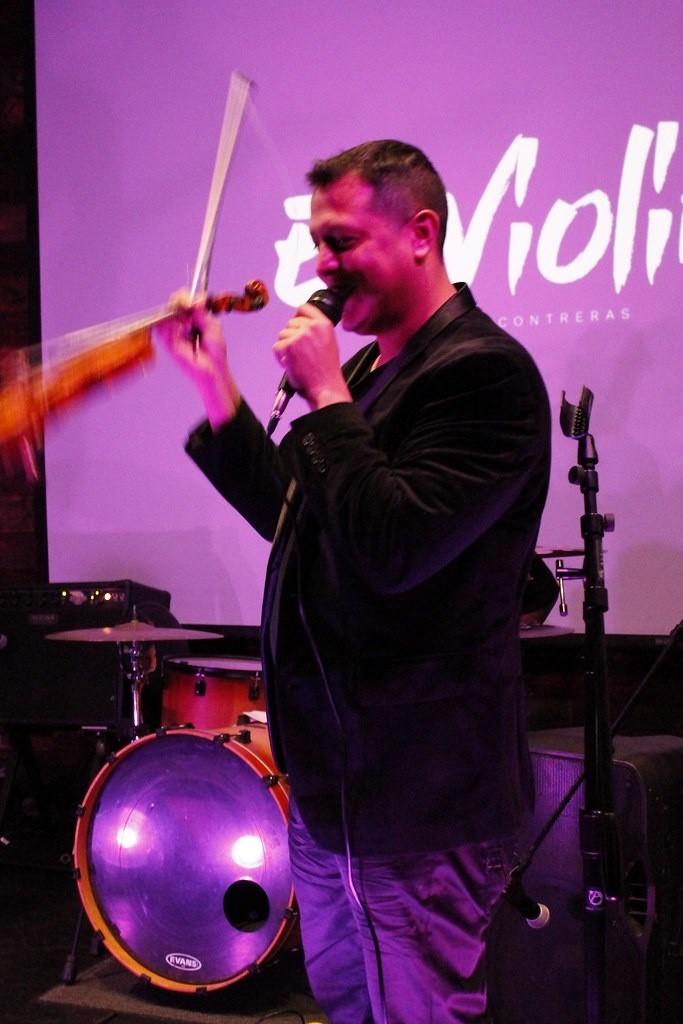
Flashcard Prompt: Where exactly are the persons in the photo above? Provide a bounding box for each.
[154,137,553,1024]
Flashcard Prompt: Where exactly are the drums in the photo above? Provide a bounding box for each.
[69,719,301,998]
[157,652,269,731]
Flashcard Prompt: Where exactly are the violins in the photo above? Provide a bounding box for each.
[0,278,270,481]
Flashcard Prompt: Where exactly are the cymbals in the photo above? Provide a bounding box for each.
[518,624,575,639]
[533,547,608,558]
[43,621,225,642]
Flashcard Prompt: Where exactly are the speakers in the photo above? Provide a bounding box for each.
[482,724,683,1024]
[0,580,170,730]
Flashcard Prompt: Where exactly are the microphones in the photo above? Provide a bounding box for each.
[500,880,551,930]
[268,289,344,434]
[0,634,7,649]
